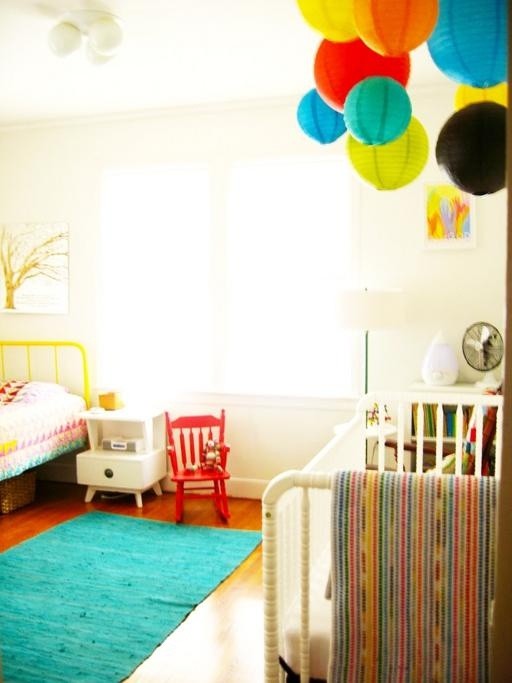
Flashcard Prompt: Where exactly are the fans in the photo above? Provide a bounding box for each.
[460,321,504,390]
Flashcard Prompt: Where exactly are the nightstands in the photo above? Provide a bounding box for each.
[76,404,166,510]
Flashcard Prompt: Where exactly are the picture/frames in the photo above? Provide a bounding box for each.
[419,177,477,250]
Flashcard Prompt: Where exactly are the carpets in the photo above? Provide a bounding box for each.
[1,509,263,682]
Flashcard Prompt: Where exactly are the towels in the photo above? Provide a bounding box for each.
[328,469,492,682]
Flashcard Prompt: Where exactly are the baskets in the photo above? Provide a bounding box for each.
[0,466,41,513]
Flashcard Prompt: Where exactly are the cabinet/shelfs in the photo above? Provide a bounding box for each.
[405,393,502,475]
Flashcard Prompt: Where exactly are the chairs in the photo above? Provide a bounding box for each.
[164,408,232,523]
[365,390,498,474]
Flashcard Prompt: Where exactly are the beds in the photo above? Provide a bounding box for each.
[0,339,90,479]
[260,390,512,683]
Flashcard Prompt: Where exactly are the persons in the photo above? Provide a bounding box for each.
[191,440,223,474]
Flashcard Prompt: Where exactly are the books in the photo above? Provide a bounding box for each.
[412,402,476,437]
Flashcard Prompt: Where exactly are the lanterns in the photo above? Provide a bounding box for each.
[295,0,510,196]
